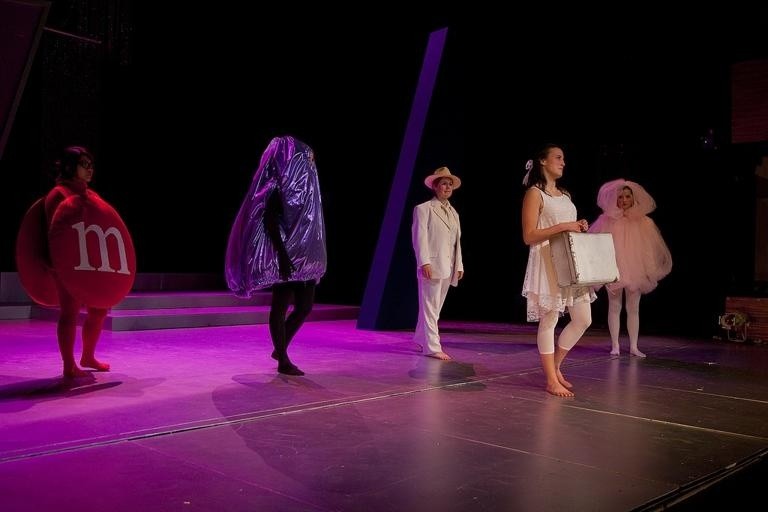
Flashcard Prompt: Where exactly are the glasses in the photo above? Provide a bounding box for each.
[78,160,96,171]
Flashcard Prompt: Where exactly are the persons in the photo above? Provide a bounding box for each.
[13,143,137,380]
[586,177,673,360]
[221,135,335,375]
[409,165,466,362]
[517,144,598,399]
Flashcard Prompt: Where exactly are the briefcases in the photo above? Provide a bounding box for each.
[549,229,620,288]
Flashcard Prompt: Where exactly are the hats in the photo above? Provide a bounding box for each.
[424,167,461,190]
